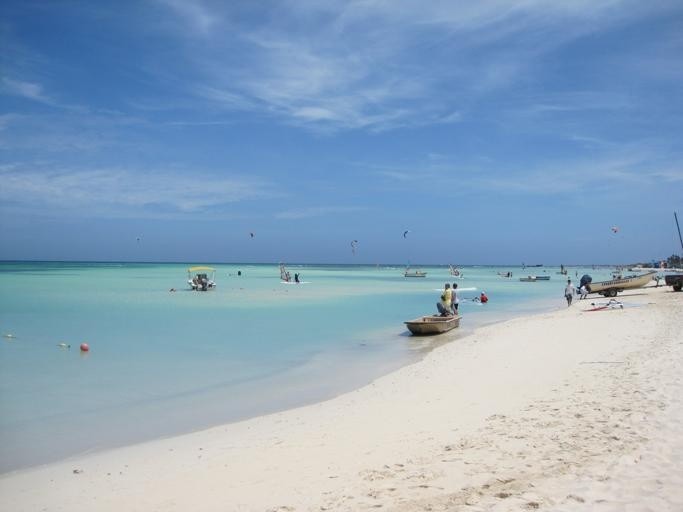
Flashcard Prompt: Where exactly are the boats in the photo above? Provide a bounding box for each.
[404,313,462,335]
[188,266,216,291]
[405,264,658,297]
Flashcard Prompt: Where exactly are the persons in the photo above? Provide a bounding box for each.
[579,282,588,300]
[437,283,452,315]
[480,292,488,302]
[563,280,575,307]
[286,271,292,282]
[294,272,300,284]
[451,284,459,314]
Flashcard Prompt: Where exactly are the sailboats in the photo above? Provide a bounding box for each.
[280,262,312,284]
